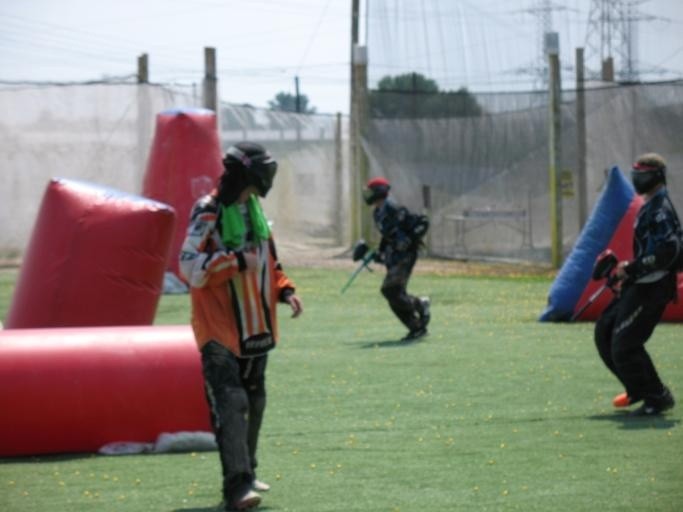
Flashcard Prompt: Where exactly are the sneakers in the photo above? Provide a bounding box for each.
[419,296,430,327]
[251,478,271,492]
[225,488,260,510]
[632,386,674,416]
[401,326,429,343]
[613,392,642,407]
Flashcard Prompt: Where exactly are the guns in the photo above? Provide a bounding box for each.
[339,239,384,295]
[569,249,625,323]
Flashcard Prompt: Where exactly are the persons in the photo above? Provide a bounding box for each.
[593,155,683,415]
[179,142,302,508]
[361,178,430,340]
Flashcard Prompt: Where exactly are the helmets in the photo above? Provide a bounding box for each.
[223,143,277,198]
[362,179,390,205]
[630,154,666,194]
